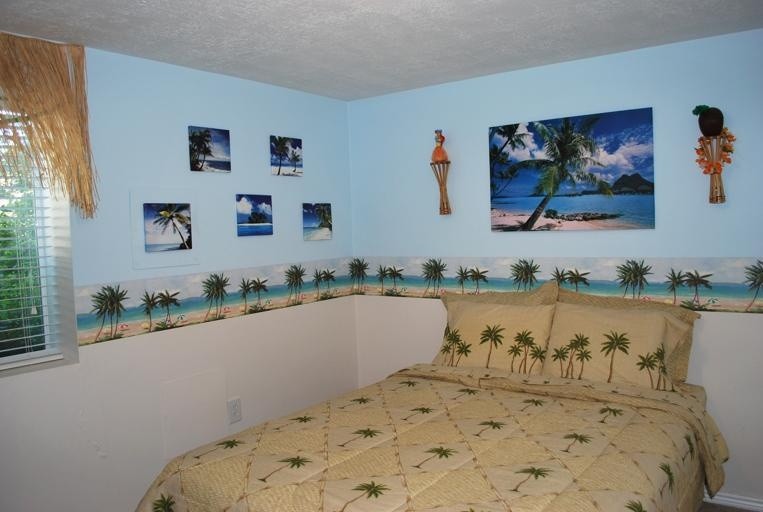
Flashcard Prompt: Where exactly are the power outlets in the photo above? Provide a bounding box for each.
[227,397,242,424]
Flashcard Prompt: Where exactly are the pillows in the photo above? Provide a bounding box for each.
[430,280,559,377]
[540,288,700,393]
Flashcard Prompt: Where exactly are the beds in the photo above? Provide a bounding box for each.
[135,362,707,512]
